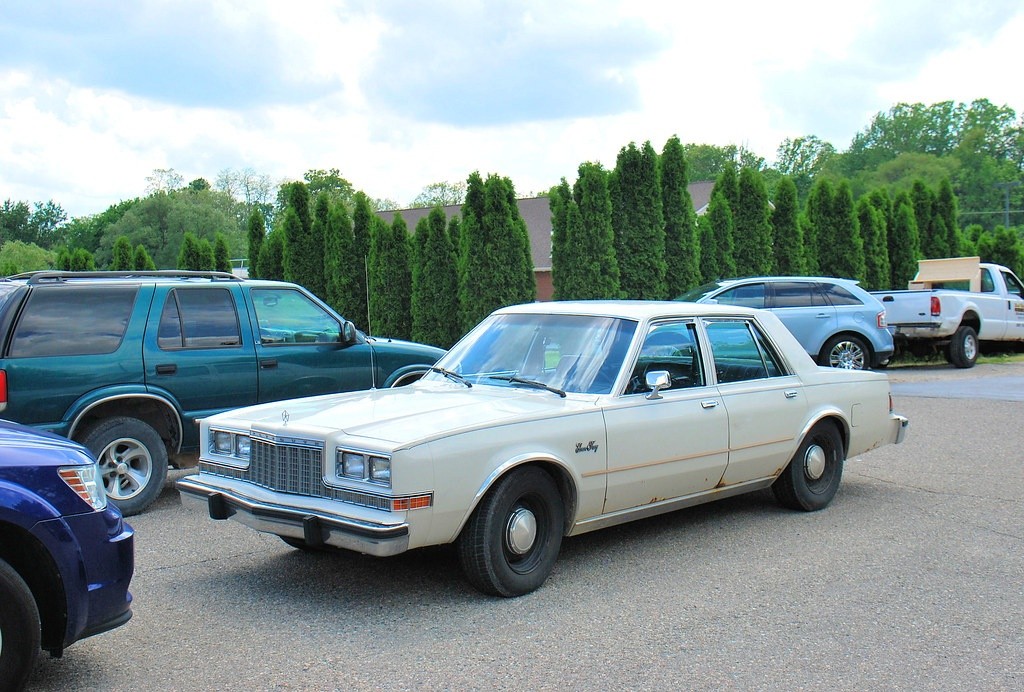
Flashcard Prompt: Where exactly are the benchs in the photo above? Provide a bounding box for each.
[636,361,775,382]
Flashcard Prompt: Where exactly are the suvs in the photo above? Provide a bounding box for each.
[0,271,462,519]
[645,274,895,372]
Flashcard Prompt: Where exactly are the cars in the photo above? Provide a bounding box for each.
[0,417,135,692]
[175,298,909,598]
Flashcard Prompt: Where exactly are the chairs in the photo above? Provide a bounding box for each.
[636,364,694,391]
[553,355,610,381]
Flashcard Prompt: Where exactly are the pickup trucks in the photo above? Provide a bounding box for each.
[866,262,1024,368]
